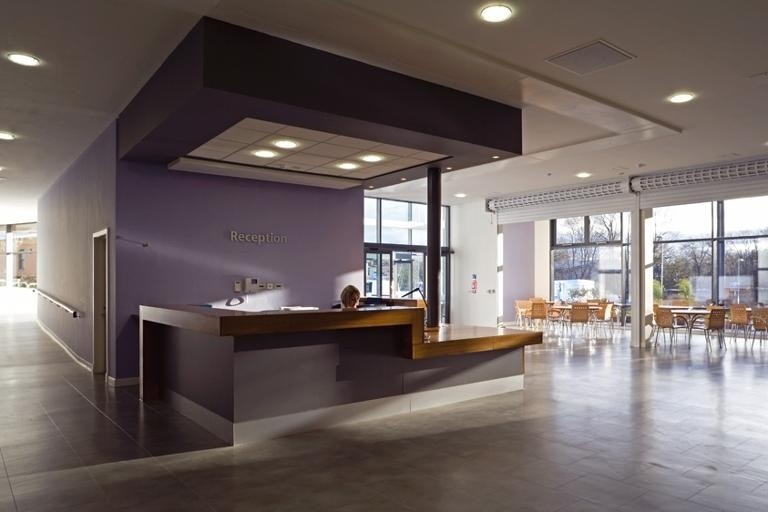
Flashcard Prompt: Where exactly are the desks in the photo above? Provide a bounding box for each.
[550,306,603,341]
[671,310,711,351]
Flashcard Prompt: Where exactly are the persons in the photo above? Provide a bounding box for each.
[343,290,360,309]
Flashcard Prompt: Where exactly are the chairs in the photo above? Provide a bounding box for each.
[654,309,690,354]
[529,298,544,302]
[589,302,615,340]
[728,304,752,345]
[560,304,589,339]
[751,308,768,342]
[528,303,549,331]
[688,309,728,355]
[513,300,534,329]
